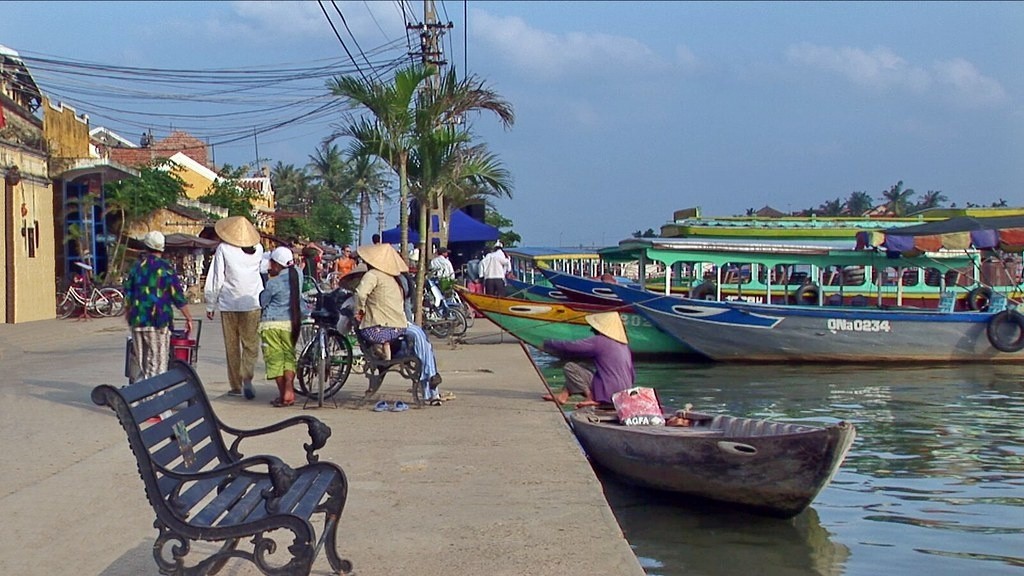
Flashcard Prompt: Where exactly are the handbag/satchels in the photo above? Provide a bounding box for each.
[611,388,666,426]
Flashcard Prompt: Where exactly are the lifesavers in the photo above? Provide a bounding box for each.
[968,286,993,312]
[794,283,825,305]
[691,281,722,301]
[986,309,1024,353]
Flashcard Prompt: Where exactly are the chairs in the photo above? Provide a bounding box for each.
[788,272,940,286]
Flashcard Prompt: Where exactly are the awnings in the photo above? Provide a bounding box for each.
[125,233,218,250]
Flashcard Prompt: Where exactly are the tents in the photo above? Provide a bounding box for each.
[381,199,505,255]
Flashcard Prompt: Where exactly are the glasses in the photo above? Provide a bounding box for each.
[345,250,352,254]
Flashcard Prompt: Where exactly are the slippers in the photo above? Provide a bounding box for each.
[243,384,256,400]
[391,401,408,412]
[227,389,244,396]
[373,400,389,412]
[271,397,294,408]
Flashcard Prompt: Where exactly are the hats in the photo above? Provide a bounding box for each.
[214,215,261,247]
[437,247,452,255]
[392,246,409,273]
[263,246,294,267]
[584,312,629,344]
[143,230,165,251]
[356,243,400,276]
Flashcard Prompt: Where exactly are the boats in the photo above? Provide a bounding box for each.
[570,406,856,521]
[450,208,1023,364]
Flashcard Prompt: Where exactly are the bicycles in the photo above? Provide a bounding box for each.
[408,269,475,337]
[293,272,368,411]
[55,252,126,319]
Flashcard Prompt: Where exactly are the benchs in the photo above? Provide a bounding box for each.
[90,359,335,576]
[619,425,722,434]
[341,308,422,408]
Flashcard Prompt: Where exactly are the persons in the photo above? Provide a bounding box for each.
[125,230,194,424]
[542,311,635,410]
[203,215,264,399]
[336,242,442,407]
[259,246,303,407]
[260,234,512,330]
